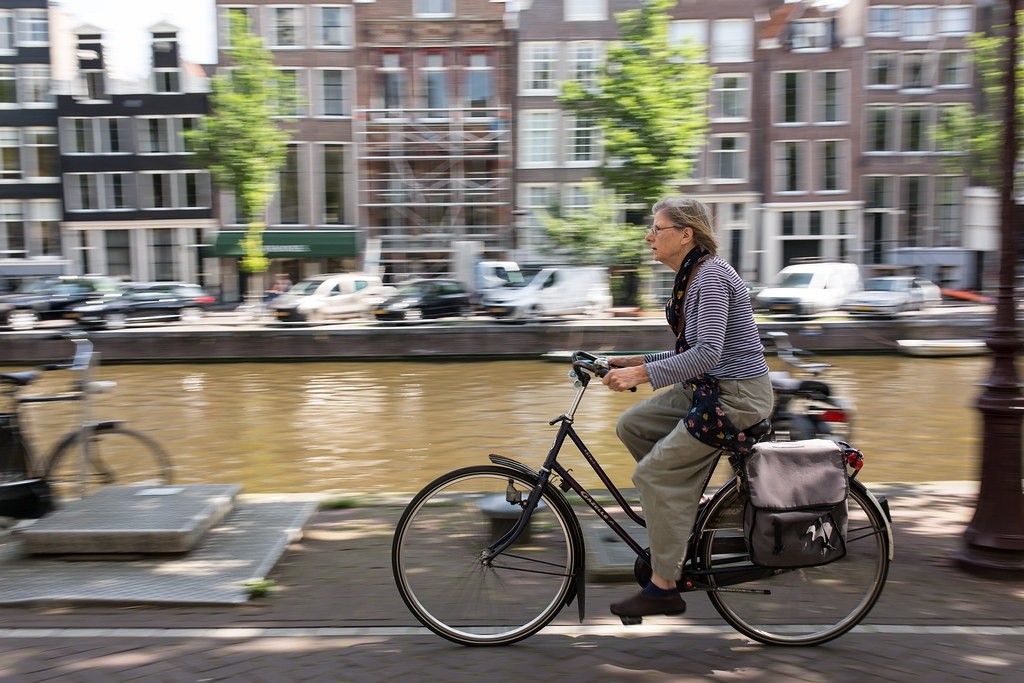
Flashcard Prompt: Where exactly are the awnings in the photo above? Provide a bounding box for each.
[199,226,358,259]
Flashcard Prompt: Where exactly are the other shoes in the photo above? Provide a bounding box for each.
[609,592,688,618]
[696,495,725,528]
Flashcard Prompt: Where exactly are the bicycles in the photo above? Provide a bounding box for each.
[389,347,900,646]
[762,328,846,444]
[1,324,176,526]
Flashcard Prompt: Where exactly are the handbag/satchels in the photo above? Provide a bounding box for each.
[739,437,850,570]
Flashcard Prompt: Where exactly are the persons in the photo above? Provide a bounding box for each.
[602,195,775,617]
[272,273,293,294]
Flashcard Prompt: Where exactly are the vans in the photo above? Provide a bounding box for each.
[750,261,864,315]
[479,259,617,326]
[266,273,397,325]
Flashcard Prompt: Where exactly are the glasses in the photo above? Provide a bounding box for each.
[650,224,680,236]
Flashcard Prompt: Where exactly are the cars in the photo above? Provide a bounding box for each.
[0,272,117,332]
[373,279,486,323]
[840,276,944,317]
[58,274,215,335]
